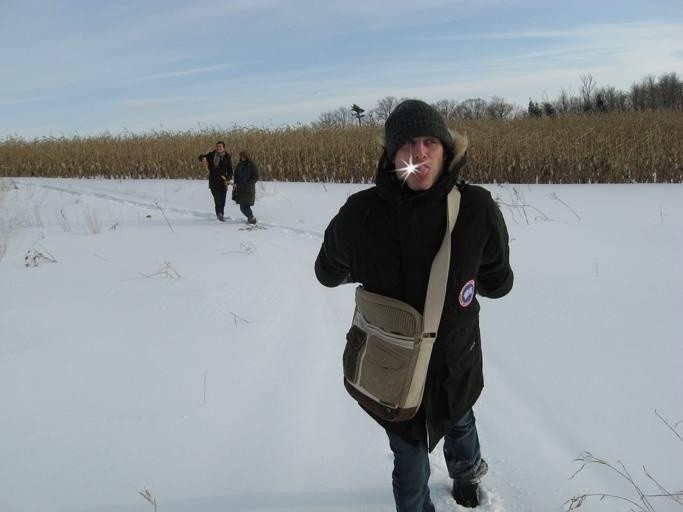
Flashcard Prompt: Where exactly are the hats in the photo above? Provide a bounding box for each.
[384,99,453,163]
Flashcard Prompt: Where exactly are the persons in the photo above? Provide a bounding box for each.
[231,151,258,224]
[314,99,513,511]
[198,141,232,222]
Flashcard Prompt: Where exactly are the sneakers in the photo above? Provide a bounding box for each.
[452,480,480,508]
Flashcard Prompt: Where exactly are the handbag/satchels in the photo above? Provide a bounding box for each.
[343,284,430,423]
